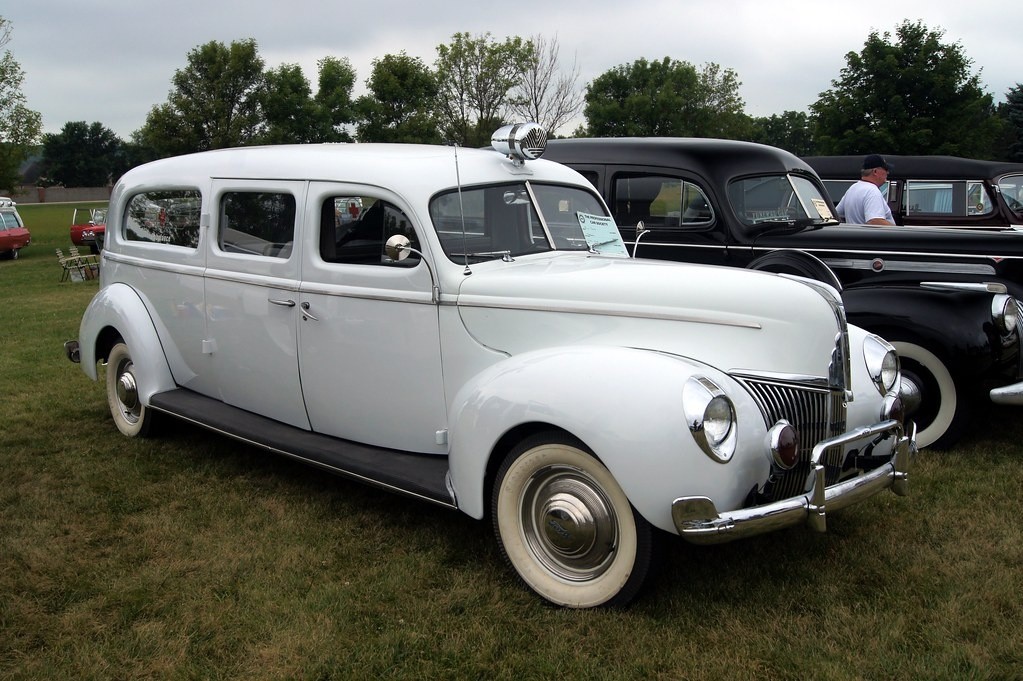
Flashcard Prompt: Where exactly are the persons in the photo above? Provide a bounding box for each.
[831,153,896,227]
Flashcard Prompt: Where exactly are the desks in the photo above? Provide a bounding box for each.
[60,254,100,282]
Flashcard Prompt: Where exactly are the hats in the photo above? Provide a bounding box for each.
[862,154,894,170]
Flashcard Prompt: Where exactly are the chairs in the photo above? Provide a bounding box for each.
[55,247,85,282]
[69,246,94,281]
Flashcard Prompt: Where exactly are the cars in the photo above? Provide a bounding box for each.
[1,197,32,261]
[507,136,1023,458]
[795,155,1023,231]
[62,141,919,611]
